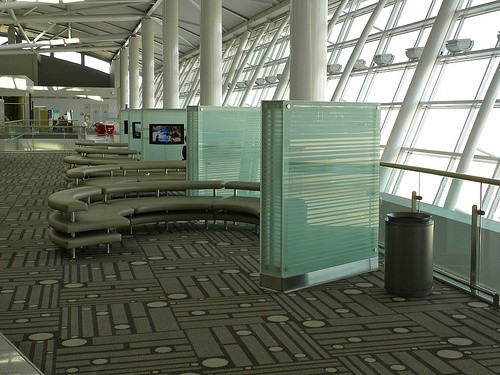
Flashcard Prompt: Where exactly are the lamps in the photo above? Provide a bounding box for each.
[373,53,394,67]
[405,46,425,60]
[264,76,275,83]
[444,38,474,55]
[327,64,342,75]
[353,59,366,69]
[244,81,251,86]
[236,82,244,88]
[277,74,280,80]
[255,78,265,85]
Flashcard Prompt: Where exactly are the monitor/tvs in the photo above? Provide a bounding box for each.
[132,122,141,138]
[124,120,128,135]
[149,124,184,144]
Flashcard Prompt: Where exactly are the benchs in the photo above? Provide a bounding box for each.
[48,139,261,258]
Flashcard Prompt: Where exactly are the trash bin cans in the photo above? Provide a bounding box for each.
[385,211,434,298]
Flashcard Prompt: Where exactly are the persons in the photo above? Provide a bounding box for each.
[153,126,181,143]
[182,136,187,160]
[55,117,61,126]
